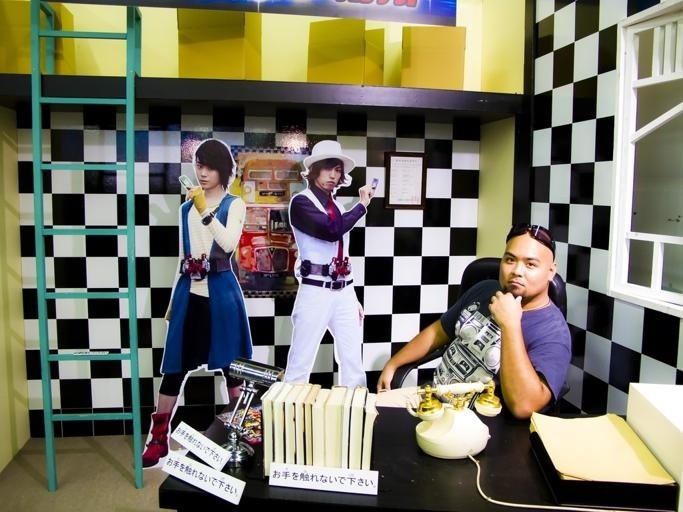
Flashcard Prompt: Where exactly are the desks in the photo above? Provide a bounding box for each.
[159,396,678,511]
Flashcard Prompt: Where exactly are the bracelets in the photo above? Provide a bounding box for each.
[201,207,219,225]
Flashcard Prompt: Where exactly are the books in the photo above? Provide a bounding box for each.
[260,381,377,477]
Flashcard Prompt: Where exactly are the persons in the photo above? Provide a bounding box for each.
[141,139,252,467]
[376,221,571,419]
[281,139,375,387]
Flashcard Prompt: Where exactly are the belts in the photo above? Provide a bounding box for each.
[301,277,353,290]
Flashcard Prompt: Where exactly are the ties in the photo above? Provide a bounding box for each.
[328,197,342,261]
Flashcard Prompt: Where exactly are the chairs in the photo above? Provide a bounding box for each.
[390,258,570,408]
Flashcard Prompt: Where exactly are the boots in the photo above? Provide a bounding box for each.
[142,412,171,466]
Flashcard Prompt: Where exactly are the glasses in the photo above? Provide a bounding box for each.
[505,223,555,262]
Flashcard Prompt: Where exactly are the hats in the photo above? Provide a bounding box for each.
[302,140,355,175]
